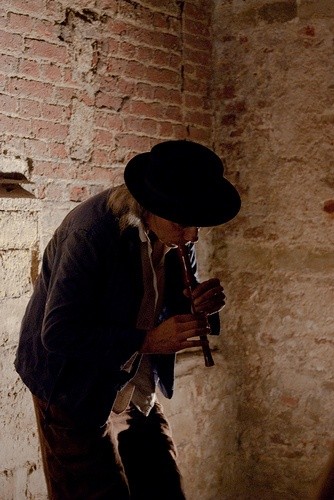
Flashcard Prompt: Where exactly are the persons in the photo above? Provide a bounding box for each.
[14,139,243,500]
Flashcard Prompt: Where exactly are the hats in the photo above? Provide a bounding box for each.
[122,140,243,227]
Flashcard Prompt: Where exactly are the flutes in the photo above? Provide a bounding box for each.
[178,243,215,367]
